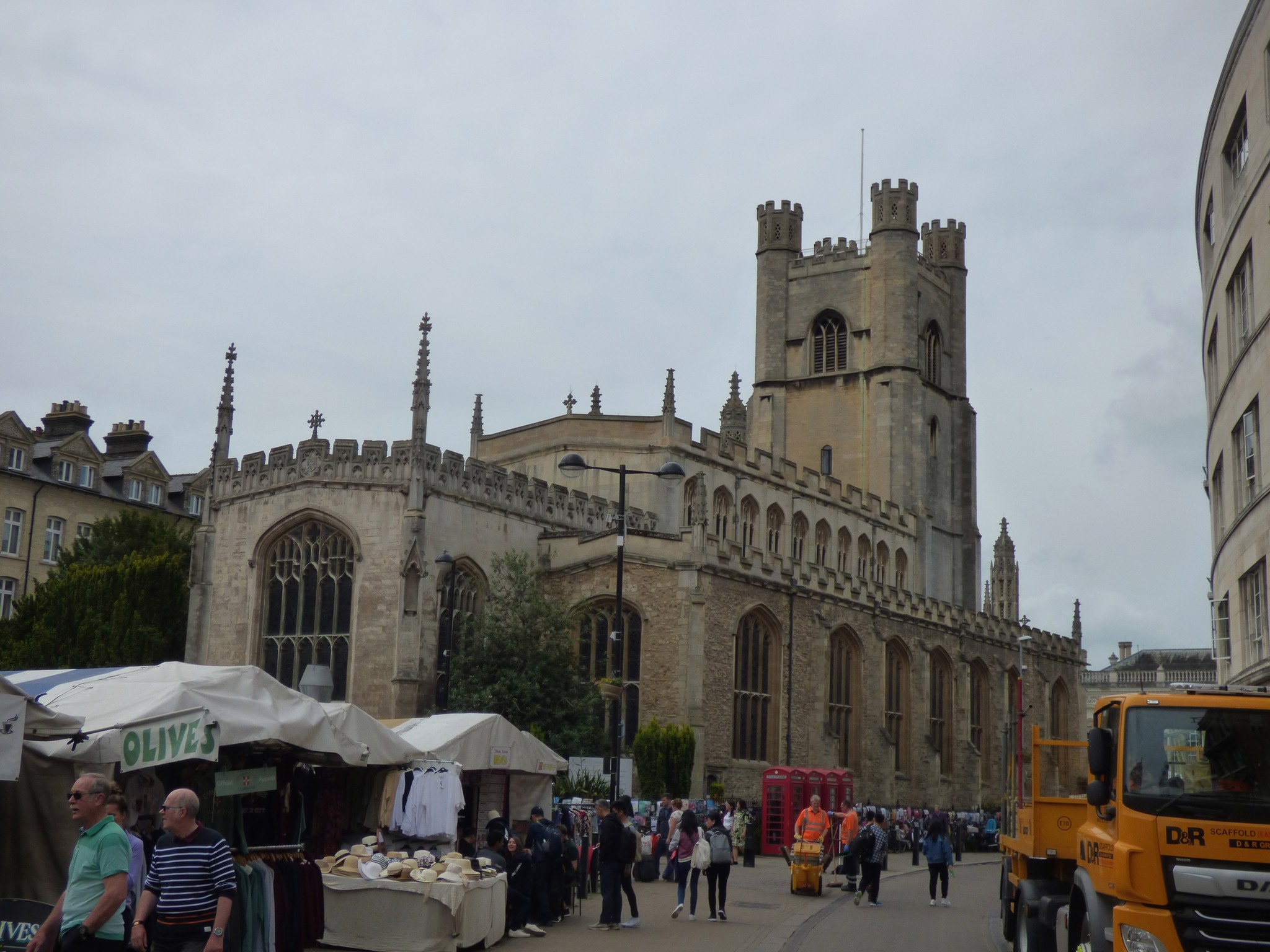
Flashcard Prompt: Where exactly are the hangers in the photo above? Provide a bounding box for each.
[553,803,596,817]
[232,843,319,874]
[398,759,448,774]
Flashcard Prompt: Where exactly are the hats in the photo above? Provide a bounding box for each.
[313,835,500,884]
[485,810,504,829]
[530,806,543,820]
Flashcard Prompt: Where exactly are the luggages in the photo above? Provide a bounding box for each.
[632,855,656,883]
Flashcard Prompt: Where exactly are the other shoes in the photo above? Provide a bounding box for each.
[508,929,531,938]
[941,898,951,906]
[708,915,717,922]
[930,899,937,906]
[552,914,562,922]
[545,920,553,927]
[589,922,609,930]
[525,923,547,936]
[608,922,619,930]
[841,885,857,892]
[689,914,695,921]
[563,908,570,917]
[853,890,863,906]
[718,909,727,920]
[869,900,882,906]
[621,917,640,928]
[671,904,683,919]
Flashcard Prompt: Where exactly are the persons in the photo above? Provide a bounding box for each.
[662,798,684,882]
[922,820,954,906]
[721,799,735,866]
[730,799,751,865]
[931,804,945,825]
[456,806,579,938]
[669,809,705,921]
[702,812,733,922]
[853,810,889,906]
[105,781,144,952]
[1113,757,1160,793]
[827,800,858,892]
[794,795,830,843]
[130,788,237,952]
[589,795,640,931]
[650,793,674,883]
[26,773,132,952]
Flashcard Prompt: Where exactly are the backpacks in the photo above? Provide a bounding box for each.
[625,821,642,862]
[604,814,637,864]
[690,826,712,872]
[706,830,733,865]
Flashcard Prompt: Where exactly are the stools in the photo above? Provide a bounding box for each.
[561,883,581,921]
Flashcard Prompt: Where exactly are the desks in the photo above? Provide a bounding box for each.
[318,868,509,952]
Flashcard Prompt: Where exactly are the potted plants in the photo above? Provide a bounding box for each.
[552,750,611,809]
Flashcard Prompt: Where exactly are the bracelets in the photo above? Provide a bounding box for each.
[833,812,836,815]
[130,921,147,931]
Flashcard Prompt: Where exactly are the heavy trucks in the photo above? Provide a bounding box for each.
[995,682,1270,952]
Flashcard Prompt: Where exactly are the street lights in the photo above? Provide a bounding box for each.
[556,452,687,794]
[1017,634,1034,684]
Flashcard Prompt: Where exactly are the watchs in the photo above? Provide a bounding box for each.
[211,928,224,936]
[78,923,89,938]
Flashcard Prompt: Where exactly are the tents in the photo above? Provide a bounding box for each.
[0,660,568,915]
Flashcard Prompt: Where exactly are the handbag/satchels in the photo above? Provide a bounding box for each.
[639,832,652,857]
[846,824,876,857]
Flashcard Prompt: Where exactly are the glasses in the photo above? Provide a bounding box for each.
[67,791,101,800]
[161,804,182,812]
